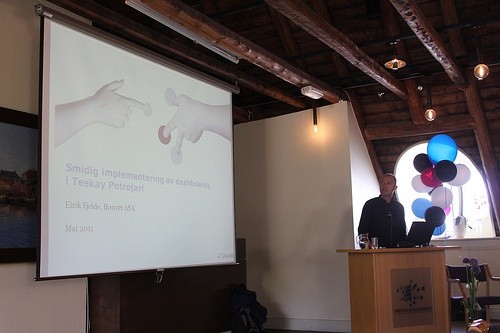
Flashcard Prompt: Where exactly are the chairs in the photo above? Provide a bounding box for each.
[445,263,500,330]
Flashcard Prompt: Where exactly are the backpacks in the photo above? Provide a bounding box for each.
[230,285,267,332]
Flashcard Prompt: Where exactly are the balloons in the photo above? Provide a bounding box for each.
[413,153,433,173]
[411,159,457,236]
[448,163,471,186]
[427,134,458,166]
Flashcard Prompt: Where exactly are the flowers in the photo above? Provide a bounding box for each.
[459,257,483,320]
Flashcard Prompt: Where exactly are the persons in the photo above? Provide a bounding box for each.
[357,173,406,248]
[54,79,233,154]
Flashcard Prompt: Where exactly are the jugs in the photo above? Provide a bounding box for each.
[356,233,370,249]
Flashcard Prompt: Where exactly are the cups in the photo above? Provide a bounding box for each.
[371,238,378,249]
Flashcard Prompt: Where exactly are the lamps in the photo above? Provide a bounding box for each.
[382,36,407,71]
[124,0,240,64]
[310,101,319,135]
[473,46,490,81]
[416,71,439,124]
[300,86,324,100]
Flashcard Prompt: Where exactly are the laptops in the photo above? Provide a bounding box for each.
[386,222,437,248]
